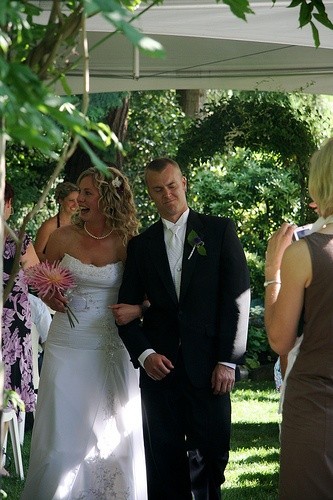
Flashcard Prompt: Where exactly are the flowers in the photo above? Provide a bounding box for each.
[3,390,26,413]
[23,259,79,329]
[112,177,121,188]
[187,229,208,260]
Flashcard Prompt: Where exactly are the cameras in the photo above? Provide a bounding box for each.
[293,223,314,242]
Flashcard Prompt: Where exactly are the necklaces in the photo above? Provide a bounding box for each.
[83,220,117,239]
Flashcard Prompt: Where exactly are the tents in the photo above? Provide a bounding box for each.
[10,0,333,94]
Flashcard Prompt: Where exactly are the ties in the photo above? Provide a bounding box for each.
[166,225,183,283]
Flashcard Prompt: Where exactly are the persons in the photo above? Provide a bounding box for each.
[0,182,40,430]
[113,158,251,500]
[265,137,333,500]
[20,167,147,500]
[35,180,81,262]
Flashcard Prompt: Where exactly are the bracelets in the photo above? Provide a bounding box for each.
[263,280,281,287]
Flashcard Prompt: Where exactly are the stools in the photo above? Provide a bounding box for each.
[0,410,25,480]
